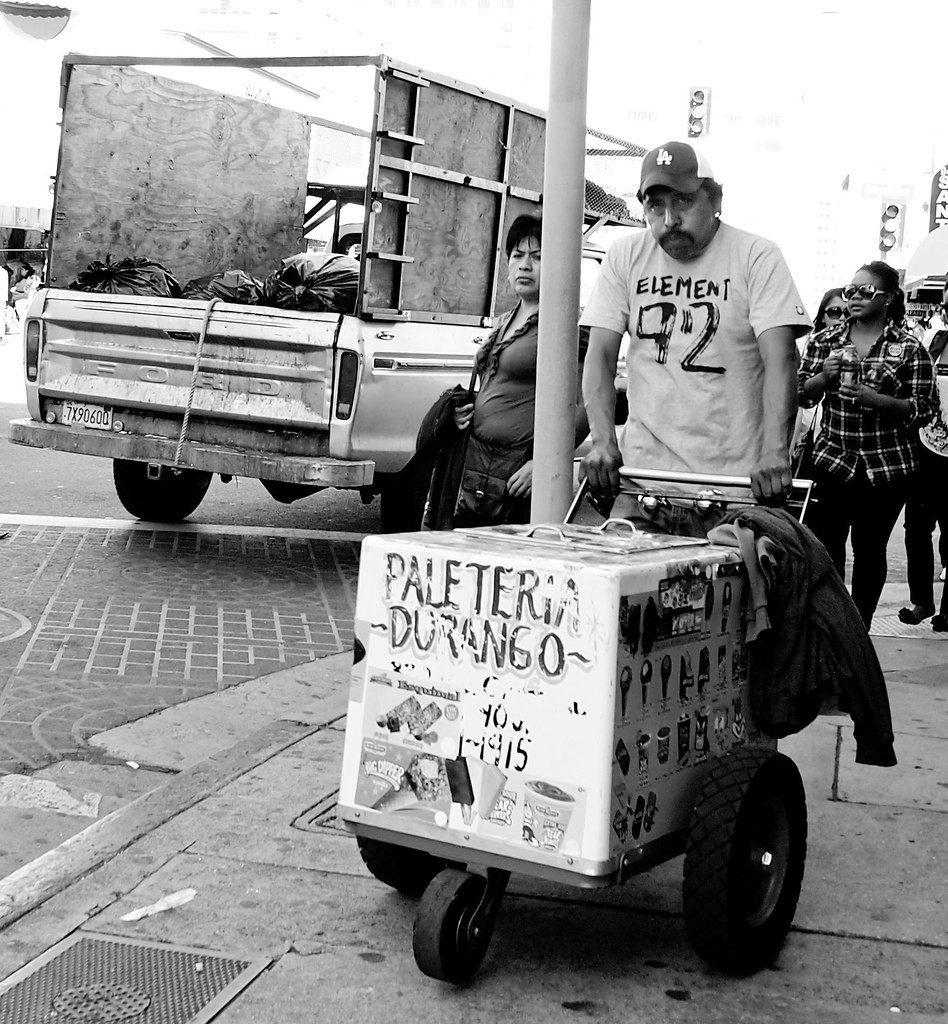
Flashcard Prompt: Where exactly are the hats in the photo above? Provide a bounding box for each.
[641,141,714,200]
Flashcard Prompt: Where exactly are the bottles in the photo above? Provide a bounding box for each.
[862,363,882,412]
[840,345,859,395]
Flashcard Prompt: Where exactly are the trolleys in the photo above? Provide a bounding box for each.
[334,465,818,982]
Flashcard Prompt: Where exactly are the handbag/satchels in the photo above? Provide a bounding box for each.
[787,430,814,499]
[452,436,529,524]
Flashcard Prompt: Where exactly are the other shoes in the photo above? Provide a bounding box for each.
[940,567,946,580]
[931,614,948,632]
[898,603,936,625]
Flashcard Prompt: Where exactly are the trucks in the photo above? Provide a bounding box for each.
[9,54,645,529]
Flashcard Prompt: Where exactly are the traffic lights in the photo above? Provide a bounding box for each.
[688,88,711,138]
[879,198,907,257]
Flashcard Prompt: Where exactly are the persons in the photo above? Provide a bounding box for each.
[0,259,42,344]
[898,279,948,633]
[789,285,850,477]
[798,262,937,634]
[577,140,814,539]
[414,214,591,527]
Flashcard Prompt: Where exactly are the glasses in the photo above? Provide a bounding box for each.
[822,306,851,319]
[841,285,889,301]
